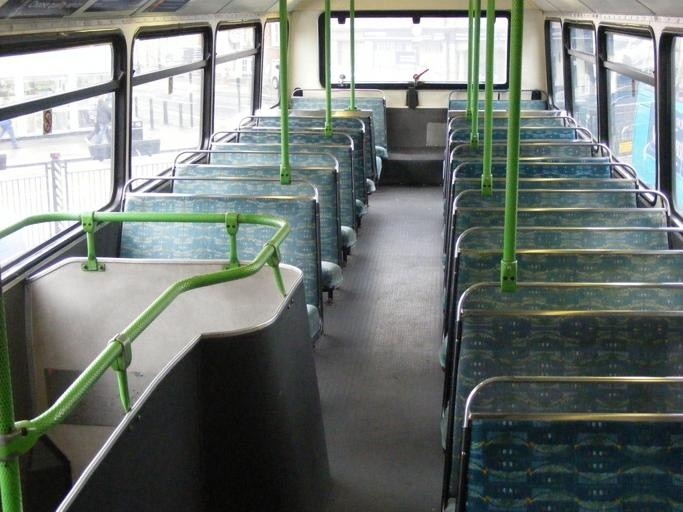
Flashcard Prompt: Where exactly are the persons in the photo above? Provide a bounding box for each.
[97,97,112,144]
[84,94,105,144]
[0,120,18,149]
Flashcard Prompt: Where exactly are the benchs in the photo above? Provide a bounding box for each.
[382,107,447,188]
